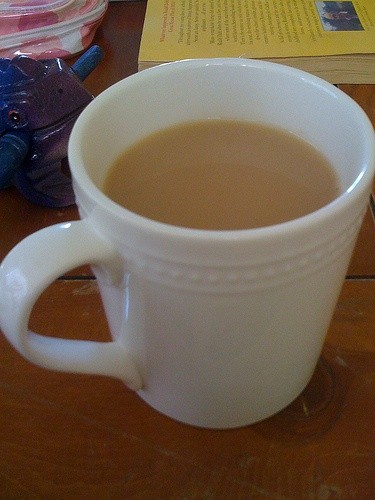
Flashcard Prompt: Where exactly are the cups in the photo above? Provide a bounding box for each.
[0,58,375,431]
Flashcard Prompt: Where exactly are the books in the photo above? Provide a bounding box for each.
[138,0,374,88]
